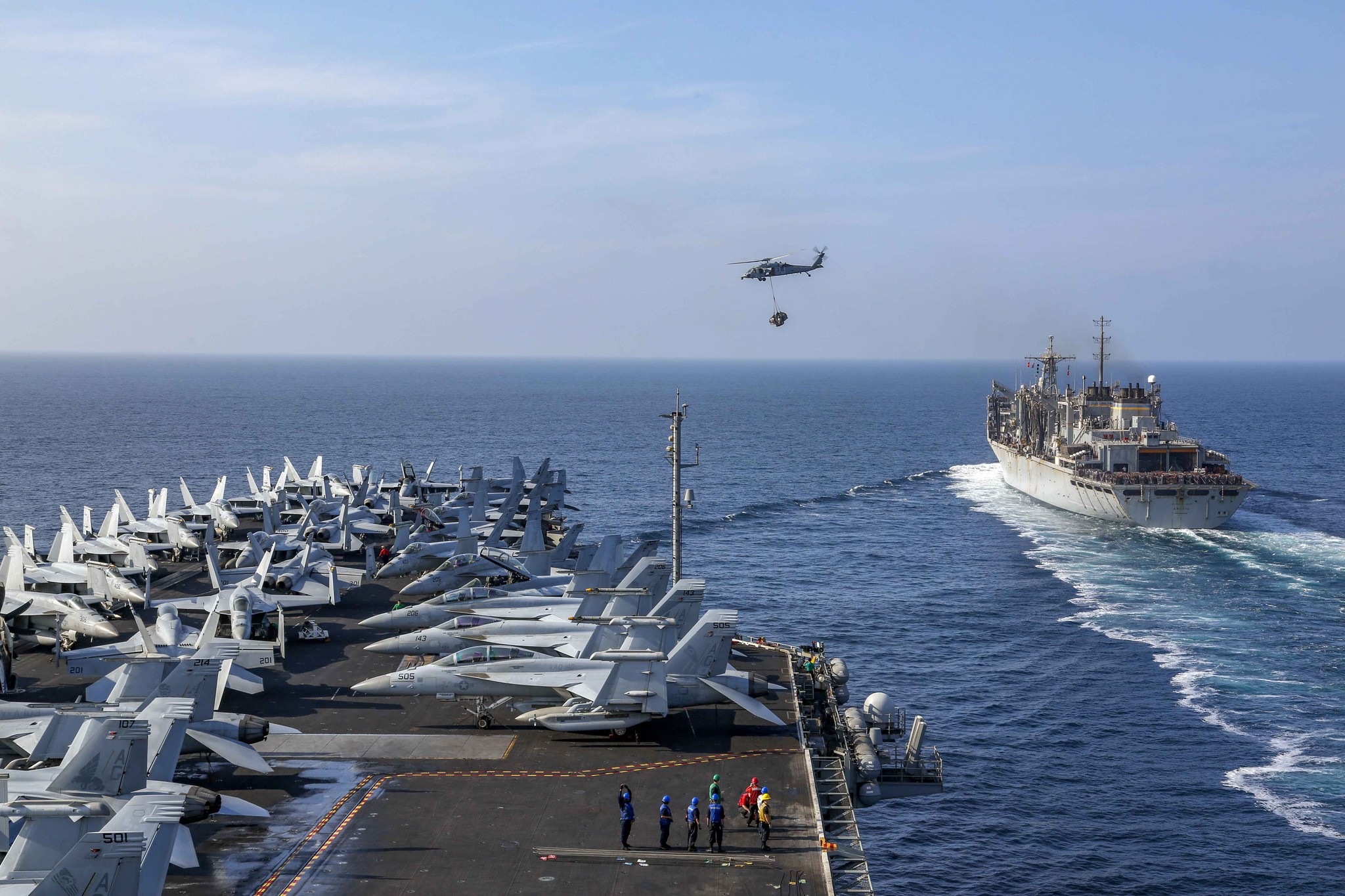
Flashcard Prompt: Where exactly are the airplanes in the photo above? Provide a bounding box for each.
[0,453,792,896]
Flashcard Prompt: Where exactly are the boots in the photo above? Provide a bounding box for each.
[759,832,771,851]
[718,846,723,853]
[747,823,750,827]
[755,824,759,827]
[741,814,745,818]
[659,842,672,850]
[621,842,631,851]
[687,846,697,852]
[706,848,713,853]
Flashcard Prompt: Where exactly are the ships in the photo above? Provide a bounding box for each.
[984,314,1257,530]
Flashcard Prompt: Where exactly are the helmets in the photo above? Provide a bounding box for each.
[662,795,671,802]
[712,794,719,800]
[691,797,700,805]
[751,777,758,784]
[762,793,771,800]
[761,787,769,793]
[622,792,630,799]
[713,774,720,781]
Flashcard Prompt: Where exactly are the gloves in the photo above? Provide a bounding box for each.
[685,817,688,822]
[699,824,702,830]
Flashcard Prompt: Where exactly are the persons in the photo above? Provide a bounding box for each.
[1122,466,1127,472]
[709,774,725,804]
[803,657,816,684]
[685,797,702,852]
[617,783,635,851]
[757,787,772,840]
[745,777,762,828]
[761,637,766,645]
[377,545,390,566]
[737,792,751,818]
[759,793,772,851]
[660,796,674,851]
[756,637,761,644]
[705,793,725,853]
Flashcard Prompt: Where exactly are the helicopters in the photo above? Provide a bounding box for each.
[727,245,829,282]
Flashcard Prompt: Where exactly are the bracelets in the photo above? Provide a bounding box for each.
[698,824,700,825]
[768,822,772,825]
